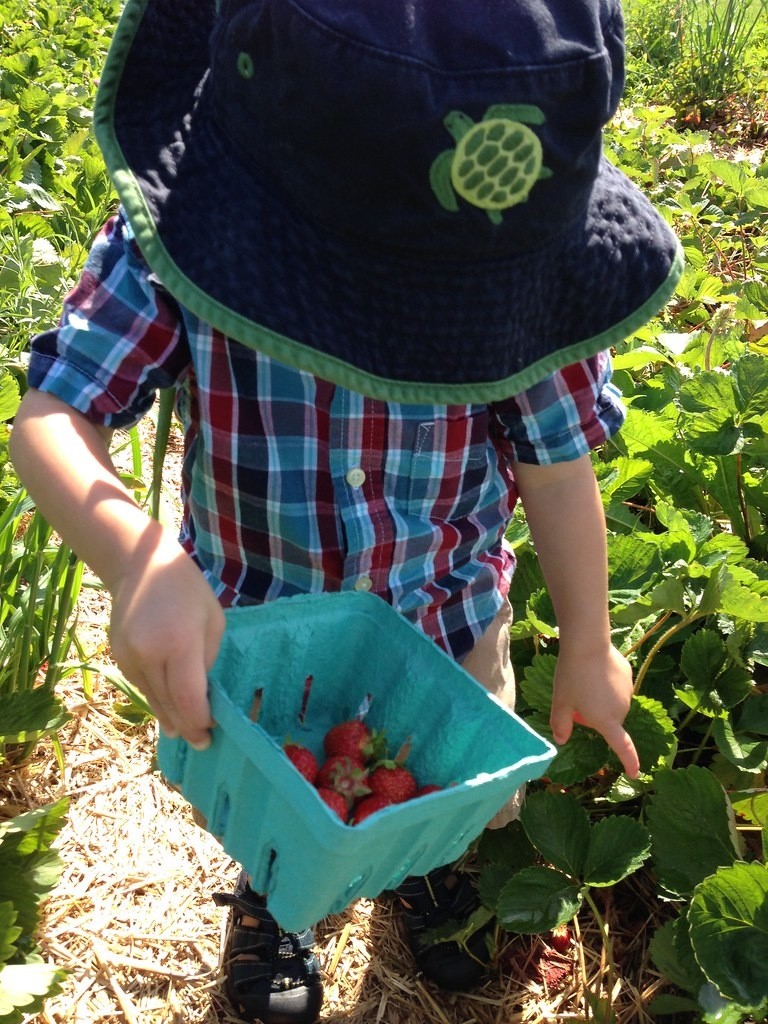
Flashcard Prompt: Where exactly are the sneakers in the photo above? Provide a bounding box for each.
[390,864,502,995]
[211,876,324,1022]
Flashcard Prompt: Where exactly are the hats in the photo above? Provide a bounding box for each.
[91,4,685,407]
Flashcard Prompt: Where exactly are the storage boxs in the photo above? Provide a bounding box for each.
[155,588,557,934]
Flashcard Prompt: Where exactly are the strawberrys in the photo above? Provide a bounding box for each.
[551,928,570,953]
[277,721,448,827]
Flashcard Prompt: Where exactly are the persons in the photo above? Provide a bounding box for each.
[8,0,682,1024]
